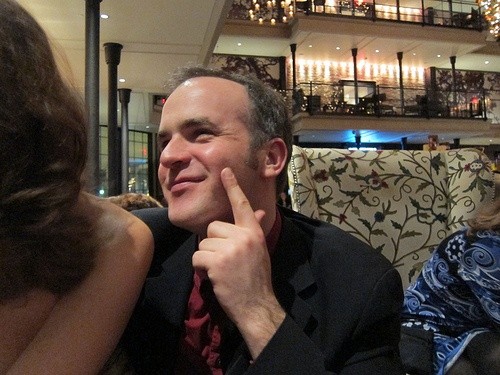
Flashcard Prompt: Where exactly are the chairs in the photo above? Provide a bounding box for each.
[286,145,495,295]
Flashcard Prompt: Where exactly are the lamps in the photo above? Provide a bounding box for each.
[244,0,294,25]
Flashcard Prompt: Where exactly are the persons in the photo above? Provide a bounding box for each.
[401,193,500,375]
[118,67,403,375]
[0,2,155,375]
